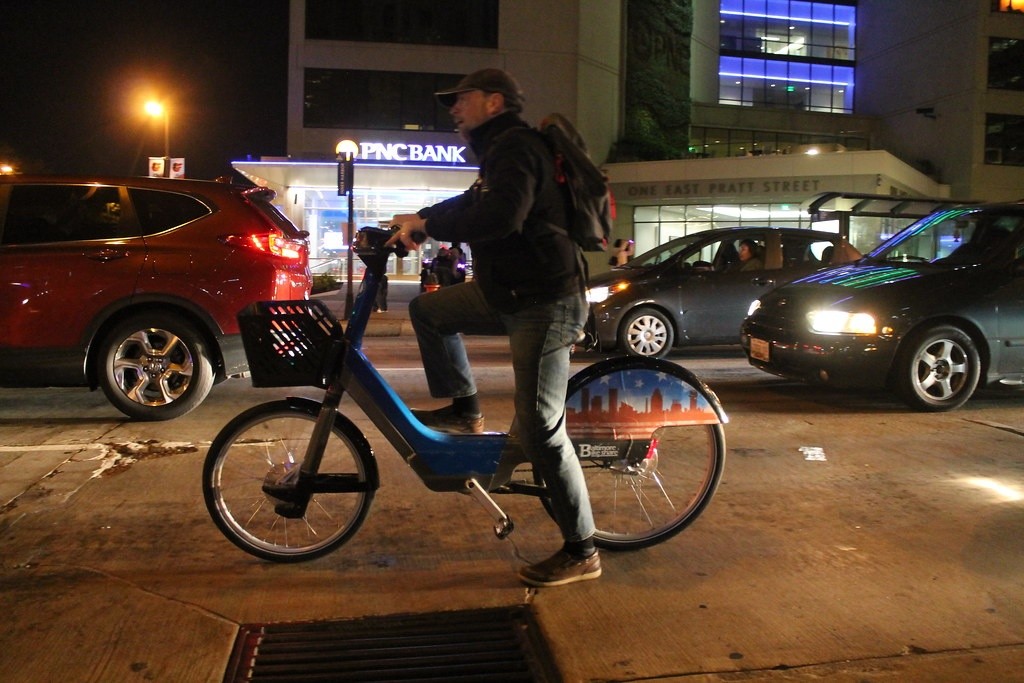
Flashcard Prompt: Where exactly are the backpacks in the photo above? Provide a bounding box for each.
[485,112,611,252]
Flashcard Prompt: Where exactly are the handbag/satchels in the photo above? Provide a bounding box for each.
[608,256,618,266]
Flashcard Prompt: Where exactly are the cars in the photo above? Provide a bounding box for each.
[0,171,313,422]
[739,199,1024,413]
[575,227,865,360]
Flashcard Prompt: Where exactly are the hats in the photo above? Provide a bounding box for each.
[434,68,526,113]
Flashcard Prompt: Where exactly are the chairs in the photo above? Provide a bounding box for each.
[820,245,833,264]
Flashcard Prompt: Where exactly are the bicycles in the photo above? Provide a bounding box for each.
[201,225,729,562]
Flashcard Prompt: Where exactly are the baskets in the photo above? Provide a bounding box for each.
[237,300,342,387]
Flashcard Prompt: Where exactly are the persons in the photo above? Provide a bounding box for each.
[348,70,601,588]
[695,240,764,273]
[430,247,464,283]
[610,239,635,268]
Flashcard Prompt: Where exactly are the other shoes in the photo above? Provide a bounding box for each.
[517,548,602,586]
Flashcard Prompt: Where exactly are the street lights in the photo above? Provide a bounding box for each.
[145,100,170,178]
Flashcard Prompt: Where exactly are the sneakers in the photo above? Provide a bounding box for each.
[409,405,485,434]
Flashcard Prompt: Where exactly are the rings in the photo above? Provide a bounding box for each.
[401,233,405,235]
